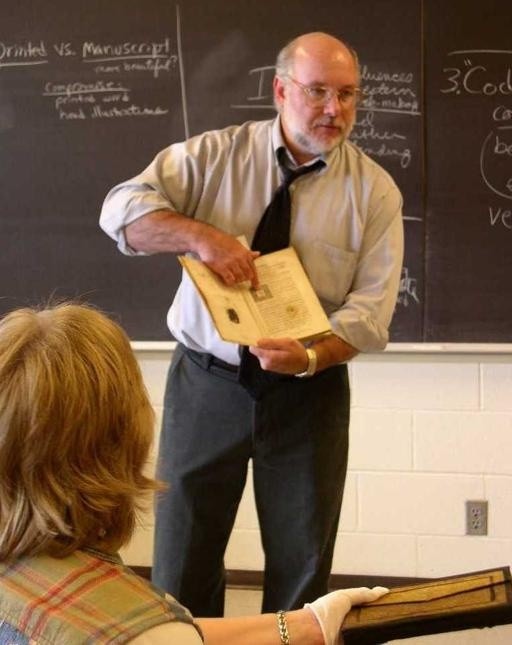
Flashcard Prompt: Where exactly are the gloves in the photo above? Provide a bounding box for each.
[303,585,389,645]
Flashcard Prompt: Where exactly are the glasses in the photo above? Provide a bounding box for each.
[285,75,359,110]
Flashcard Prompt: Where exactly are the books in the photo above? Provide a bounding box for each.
[174,247,333,348]
[339,566,512,642]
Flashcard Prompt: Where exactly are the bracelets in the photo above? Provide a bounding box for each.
[274,609,291,645]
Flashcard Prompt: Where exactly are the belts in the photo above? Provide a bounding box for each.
[211,356,236,375]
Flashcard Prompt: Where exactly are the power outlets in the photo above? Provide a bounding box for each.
[465,499,488,536]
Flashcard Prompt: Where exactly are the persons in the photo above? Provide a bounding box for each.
[99,32,405,616]
[0,300,388,645]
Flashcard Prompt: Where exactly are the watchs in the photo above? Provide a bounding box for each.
[294,346,319,381]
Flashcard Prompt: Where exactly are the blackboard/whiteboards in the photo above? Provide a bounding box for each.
[1,0,512,354]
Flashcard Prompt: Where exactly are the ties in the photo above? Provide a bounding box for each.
[237,147,327,400]
